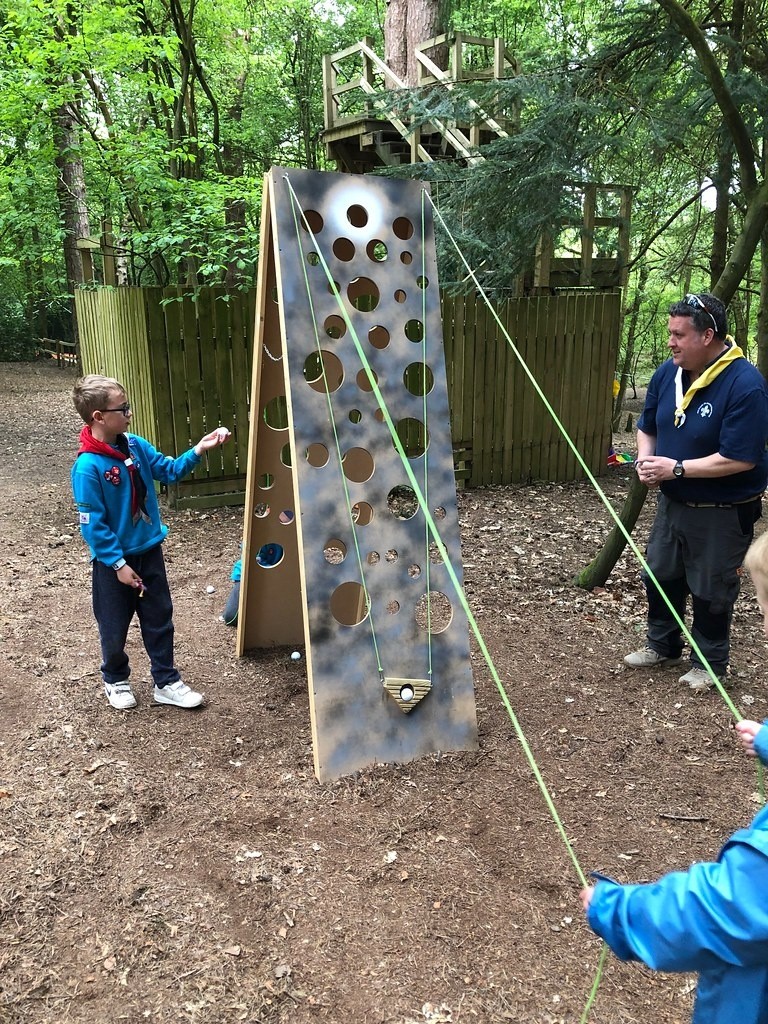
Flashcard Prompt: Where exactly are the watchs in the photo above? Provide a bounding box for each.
[111,557,127,571]
[672,460,685,479]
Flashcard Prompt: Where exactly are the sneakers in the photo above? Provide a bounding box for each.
[154,681,204,708]
[624,648,683,668]
[678,667,727,689]
[104,679,138,708]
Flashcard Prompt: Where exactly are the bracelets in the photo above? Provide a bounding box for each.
[632,459,640,469]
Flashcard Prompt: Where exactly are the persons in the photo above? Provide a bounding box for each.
[69,373,233,711]
[622,291,768,693]
[222,504,295,630]
[577,526,768,1024]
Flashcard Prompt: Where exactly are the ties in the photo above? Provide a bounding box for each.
[79,427,154,528]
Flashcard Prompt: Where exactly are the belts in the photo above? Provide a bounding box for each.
[687,493,765,508]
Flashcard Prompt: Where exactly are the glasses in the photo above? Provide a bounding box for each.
[90,403,132,423]
[681,293,718,332]
